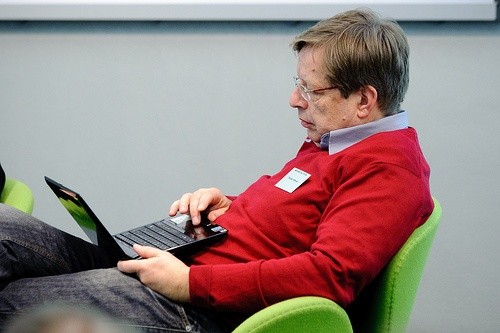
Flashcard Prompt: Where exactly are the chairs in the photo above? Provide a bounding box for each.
[227,296,354,333]
[375,195,443,333]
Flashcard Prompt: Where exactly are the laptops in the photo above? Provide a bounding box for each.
[44,176,228,267]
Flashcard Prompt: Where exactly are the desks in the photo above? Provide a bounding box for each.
[1,175,34,215]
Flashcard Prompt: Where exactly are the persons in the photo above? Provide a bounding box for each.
[0,7,435,333]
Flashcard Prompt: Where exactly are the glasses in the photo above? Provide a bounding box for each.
[293,77,337,102]
